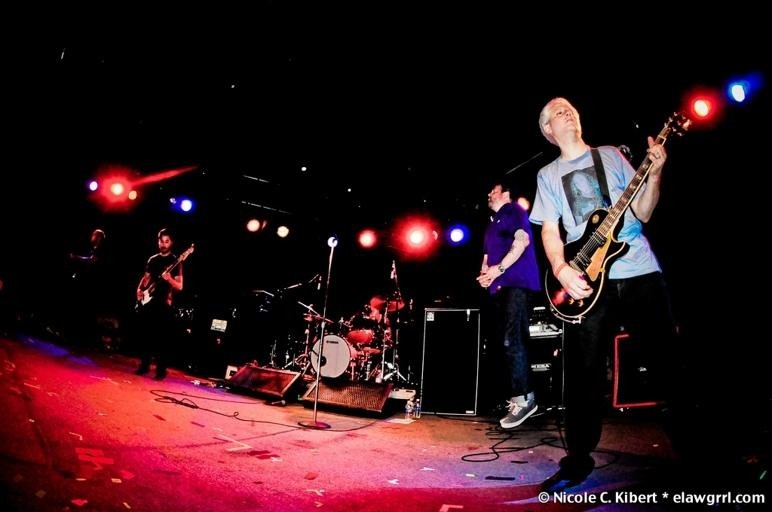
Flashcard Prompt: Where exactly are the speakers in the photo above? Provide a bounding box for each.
[228,363,302,399]
[303,375,394,413]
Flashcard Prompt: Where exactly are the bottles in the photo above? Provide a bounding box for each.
[104,342,112,358]
[405,401,413,422]
[414,398,421,419]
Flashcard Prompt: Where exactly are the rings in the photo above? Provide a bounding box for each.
[657,154,662,158]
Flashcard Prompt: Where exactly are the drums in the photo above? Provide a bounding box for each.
[347,330,382,355]
[310,333,363,380]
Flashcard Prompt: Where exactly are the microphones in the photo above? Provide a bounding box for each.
[390,260,398,287]
[327,238,336,268]
[271,400,285,406]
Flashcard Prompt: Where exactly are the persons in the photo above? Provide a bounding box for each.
[475,180,545,430]
[527,97,715,496]
[55,229,114,350]
[134,229,185,380]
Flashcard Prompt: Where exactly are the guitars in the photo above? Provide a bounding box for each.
[544,111,692,325]
[133,243,195,308]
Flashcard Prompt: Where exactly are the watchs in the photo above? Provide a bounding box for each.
[495,262,507,275]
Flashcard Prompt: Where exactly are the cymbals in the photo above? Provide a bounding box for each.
[305,314,334,324]
[297,301,321,316]
[373,301,405,312]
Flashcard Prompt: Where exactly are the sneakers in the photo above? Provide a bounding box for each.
[531,459,591,496]
[498,393,540,428]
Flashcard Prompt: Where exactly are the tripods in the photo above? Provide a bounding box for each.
[364,312,410,382]
[283,316,320,372]
